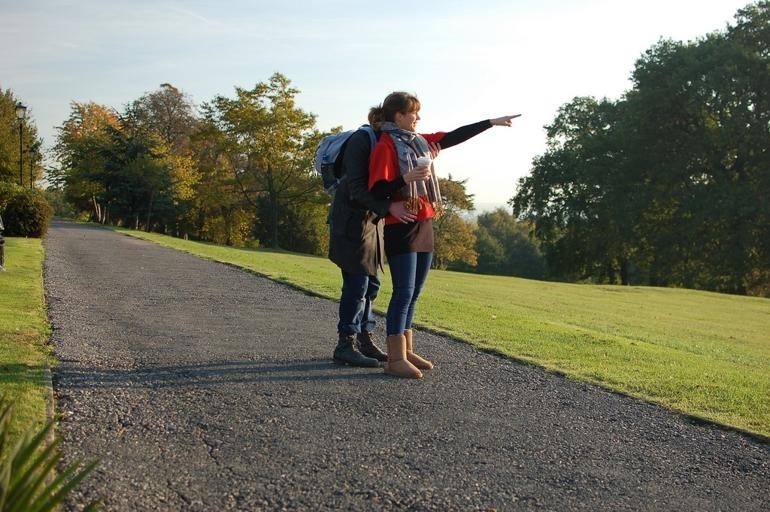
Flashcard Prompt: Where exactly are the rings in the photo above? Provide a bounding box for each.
[403,215,407,219]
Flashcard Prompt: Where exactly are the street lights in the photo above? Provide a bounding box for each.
[16,101,34,187]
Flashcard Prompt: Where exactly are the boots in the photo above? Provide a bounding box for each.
[385,333,423,380]
[331,332,380,369]
[404,328,435,370]
[357,331,388,361]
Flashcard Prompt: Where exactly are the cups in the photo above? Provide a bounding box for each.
[417,156,433,178]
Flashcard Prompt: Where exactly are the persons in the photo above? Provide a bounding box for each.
[367,91,522,379]
[328,104,442,367]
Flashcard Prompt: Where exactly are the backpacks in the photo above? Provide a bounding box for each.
[313,126,379,195]
